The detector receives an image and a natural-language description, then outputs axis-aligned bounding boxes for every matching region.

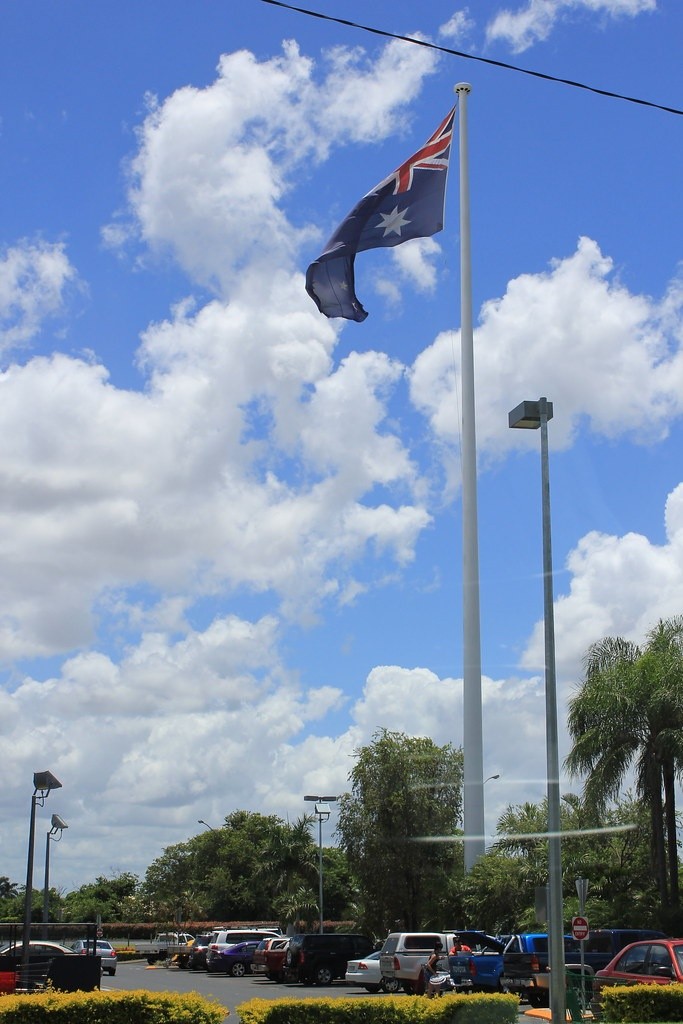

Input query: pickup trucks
[443,927,581,1001]
[251,937,298,985]
[501,930,665,1007]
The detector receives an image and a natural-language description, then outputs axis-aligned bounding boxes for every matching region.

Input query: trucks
[169,940,193,967]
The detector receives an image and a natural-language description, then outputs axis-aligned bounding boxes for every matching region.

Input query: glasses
[454,940,462,943]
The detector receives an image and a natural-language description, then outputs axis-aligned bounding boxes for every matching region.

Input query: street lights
[44,812,69,937]
[303,795,336,934]
[510,395,566,1023]
[23,770,62,991]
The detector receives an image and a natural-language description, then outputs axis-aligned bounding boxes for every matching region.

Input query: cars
[72,941,117,976]
[0,940,79,992]
[211,941,260,978]
[344,951,402,995]
[210,927,283,944]
[135,931,195,963]
[592,937,682,1005]
[187,934,214,972]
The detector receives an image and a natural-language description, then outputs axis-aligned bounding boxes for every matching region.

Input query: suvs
[256,939,289,951]
[282,935,379,987]
[380,932,498,992]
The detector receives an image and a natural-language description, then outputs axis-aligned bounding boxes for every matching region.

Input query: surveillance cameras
[52,814,69,830]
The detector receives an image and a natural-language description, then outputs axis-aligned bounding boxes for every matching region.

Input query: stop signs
[96,929,105,937]
[571,917,589,940]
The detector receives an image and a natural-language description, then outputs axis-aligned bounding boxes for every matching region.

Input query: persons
[448,937,471,995]
[424,942,445,999]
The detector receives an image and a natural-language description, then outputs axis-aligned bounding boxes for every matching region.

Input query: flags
[305,105,456,322]
